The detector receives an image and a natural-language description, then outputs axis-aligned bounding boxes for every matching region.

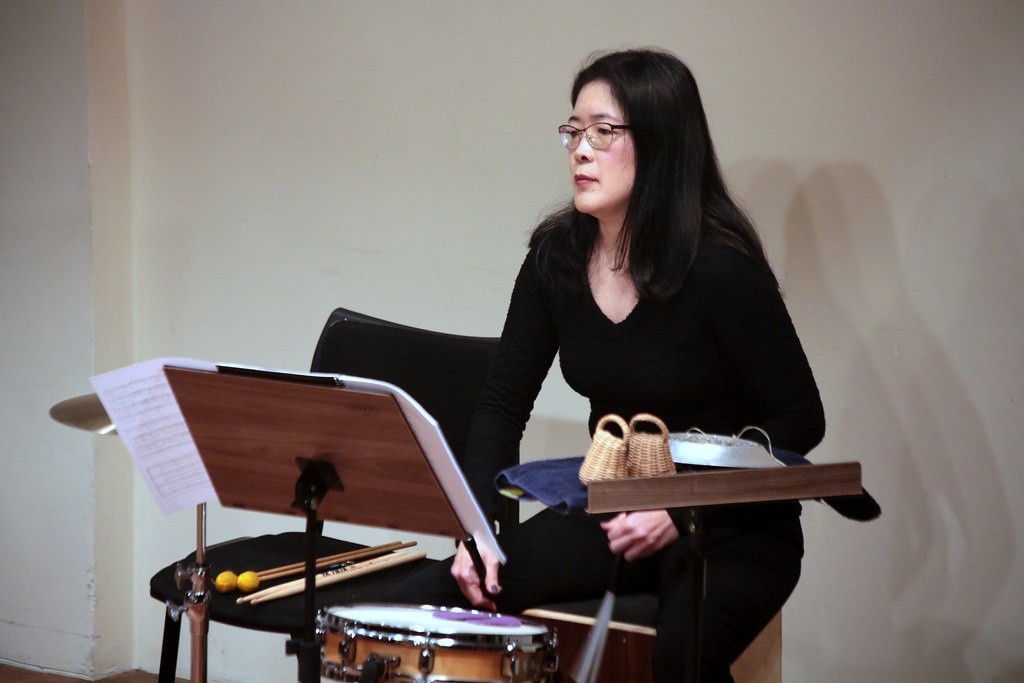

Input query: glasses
[558,123,630,150]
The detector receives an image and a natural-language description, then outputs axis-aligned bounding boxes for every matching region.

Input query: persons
[432,50,827,683]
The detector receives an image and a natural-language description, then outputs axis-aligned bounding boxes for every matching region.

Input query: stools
[523,594,784,683]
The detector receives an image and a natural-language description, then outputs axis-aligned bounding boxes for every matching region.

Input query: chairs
[150,307,501,683]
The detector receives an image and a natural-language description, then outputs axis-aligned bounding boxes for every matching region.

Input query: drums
[319,602,552,682]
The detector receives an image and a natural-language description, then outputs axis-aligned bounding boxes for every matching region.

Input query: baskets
[578,414,630,487]
[627,414,680,479]
